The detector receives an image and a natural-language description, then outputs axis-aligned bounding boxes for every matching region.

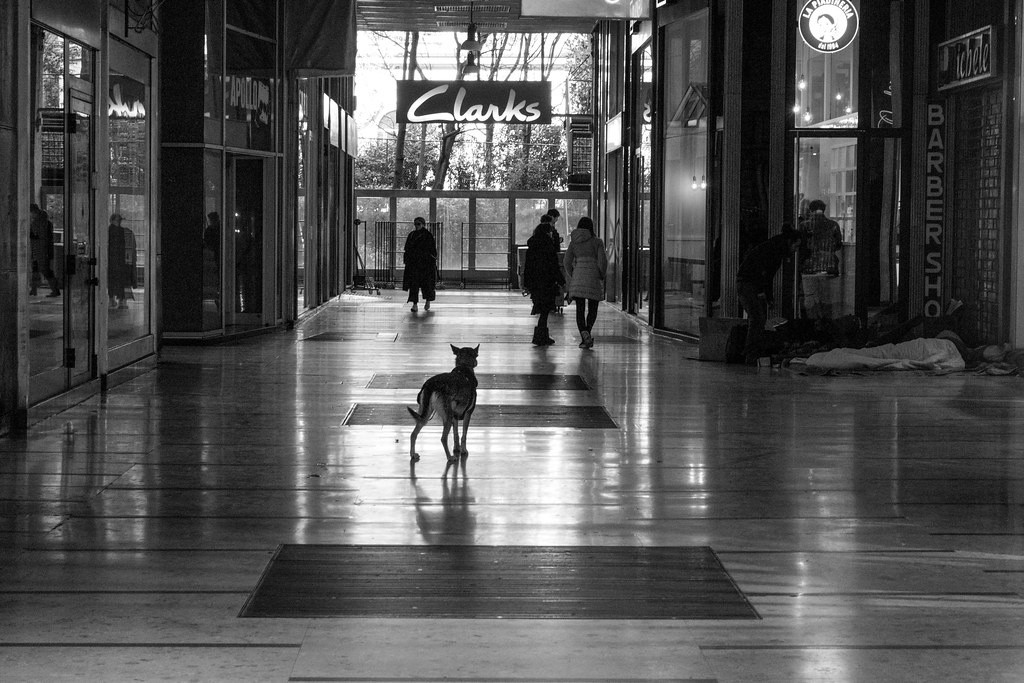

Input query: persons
[735,223,802,368]
[109,214,137,309]
[403,217,438,312]
[524,209,567,345]
[30,204,60,298]
[563,218,607,348]
[801,200,843,320]
[204,212,256,312]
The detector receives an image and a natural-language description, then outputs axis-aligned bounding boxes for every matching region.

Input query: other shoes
[46,289,62,298]
[28,288,38,295]
[424,301,431,310]
[411,304,419,312]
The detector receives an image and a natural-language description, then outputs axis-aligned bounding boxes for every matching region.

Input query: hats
[110,212,126,221]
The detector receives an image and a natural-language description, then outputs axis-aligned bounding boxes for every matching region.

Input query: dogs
[406,344,481,462]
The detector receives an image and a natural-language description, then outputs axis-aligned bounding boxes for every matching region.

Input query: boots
[532,326,556,346]
[579,341,587,348]
[580,328,594,348]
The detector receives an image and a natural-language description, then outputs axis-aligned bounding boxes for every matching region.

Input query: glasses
[414,222,422,226]
